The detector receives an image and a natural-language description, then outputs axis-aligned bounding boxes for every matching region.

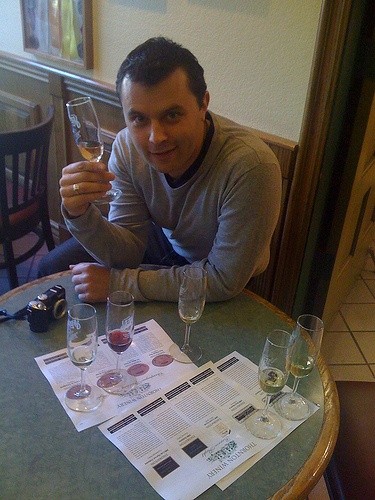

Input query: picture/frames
[20,0,94,70]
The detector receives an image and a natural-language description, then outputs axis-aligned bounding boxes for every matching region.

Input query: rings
[73,184,79,194]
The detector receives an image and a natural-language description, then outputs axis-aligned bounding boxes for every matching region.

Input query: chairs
[0,103,55,291]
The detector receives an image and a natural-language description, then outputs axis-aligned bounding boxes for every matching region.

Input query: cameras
[26,283,68,332]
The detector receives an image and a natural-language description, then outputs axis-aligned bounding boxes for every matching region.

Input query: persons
[37,37,281,303]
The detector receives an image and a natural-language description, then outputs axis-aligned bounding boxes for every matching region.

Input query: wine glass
[99,290,138,395]
[273,314,324,422]
[65,303,103,412]
[169,267,208,364]
[66,96,123,205]
[245,329,296,440]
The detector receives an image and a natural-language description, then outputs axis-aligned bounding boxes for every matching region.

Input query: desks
[0,264,340,500]
